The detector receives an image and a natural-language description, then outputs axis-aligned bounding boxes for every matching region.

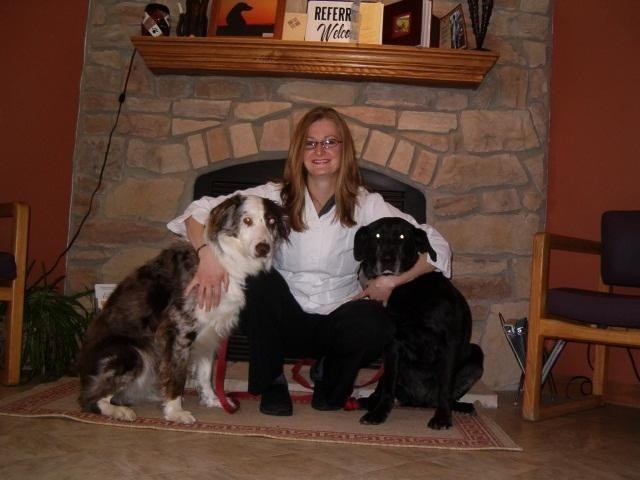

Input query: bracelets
[196,243,207,254]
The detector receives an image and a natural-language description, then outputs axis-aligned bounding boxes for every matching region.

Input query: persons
[165,104,454,416]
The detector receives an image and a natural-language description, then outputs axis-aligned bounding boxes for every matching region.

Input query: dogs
[353,216,485,431]
[76,193,294,425]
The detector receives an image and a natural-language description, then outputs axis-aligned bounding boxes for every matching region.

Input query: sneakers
[259,374,294,417]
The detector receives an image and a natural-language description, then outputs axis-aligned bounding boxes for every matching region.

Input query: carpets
[0,373,523,452]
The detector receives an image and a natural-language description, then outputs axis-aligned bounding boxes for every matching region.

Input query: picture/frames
[381,1,434,48]
[205,0,286,40]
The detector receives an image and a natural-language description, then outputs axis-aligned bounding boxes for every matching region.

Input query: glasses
[303,139,343,150]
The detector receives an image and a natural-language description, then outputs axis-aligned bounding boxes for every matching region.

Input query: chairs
[520,208,640,422]
[1,201,33,386]
